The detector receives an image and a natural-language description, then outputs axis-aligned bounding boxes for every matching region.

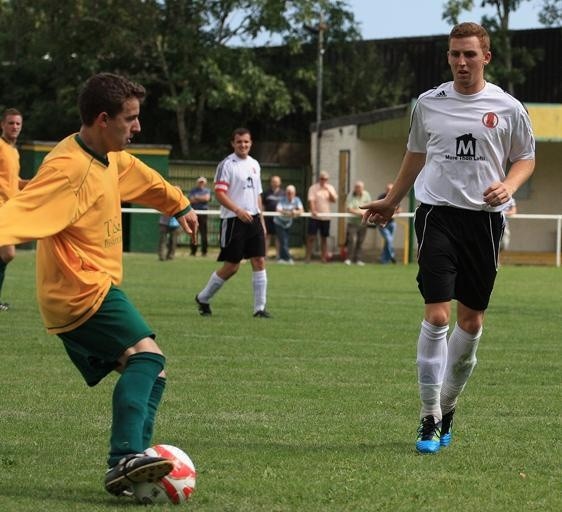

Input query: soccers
[131,444,196,505]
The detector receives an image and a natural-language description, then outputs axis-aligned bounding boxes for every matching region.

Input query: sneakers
[195,294,212,316]
[105,452,173,497]
[253,308,273,318]
[416,406,455,454]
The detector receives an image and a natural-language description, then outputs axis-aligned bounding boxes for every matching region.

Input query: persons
[188,177,211,257]
[159,183,180,262]
[262,175,285,260]
[376,182,401,265]
[342,181,371,266]
[358,23,537,455]
[304,171,337,263]
[0,73,200,498]
[194,128,273,318]
[272,184,304,264]
[0,107,30,311]
